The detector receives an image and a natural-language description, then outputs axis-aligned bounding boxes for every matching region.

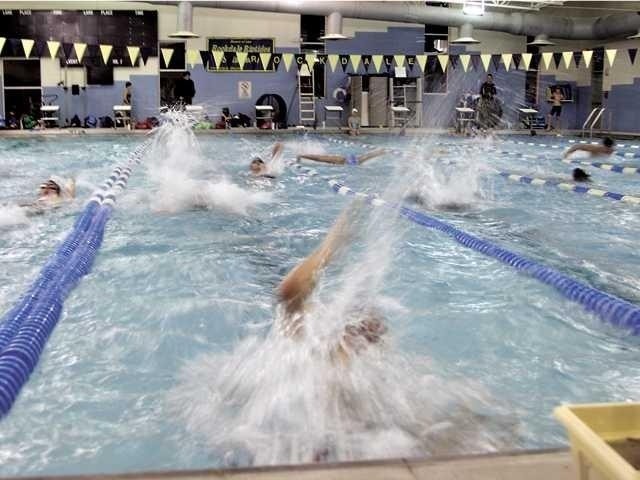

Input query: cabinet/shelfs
[351,76,423,127]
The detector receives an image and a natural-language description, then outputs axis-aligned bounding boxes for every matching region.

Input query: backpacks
[82,115,97,128]
[4,111,37,129]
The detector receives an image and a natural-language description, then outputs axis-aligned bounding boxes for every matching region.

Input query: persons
[563,137,619,159]
[458,123,495,140]
[275,211,390,364]
[241,142,285,181]
[546,87,565,132]
[296,145,398,167]
[571,169,592,183]
[123,80,132,128]
[176,72,196,105]
[348,108,362,136]
[480,74,498,101]
[18,178,77,219]
